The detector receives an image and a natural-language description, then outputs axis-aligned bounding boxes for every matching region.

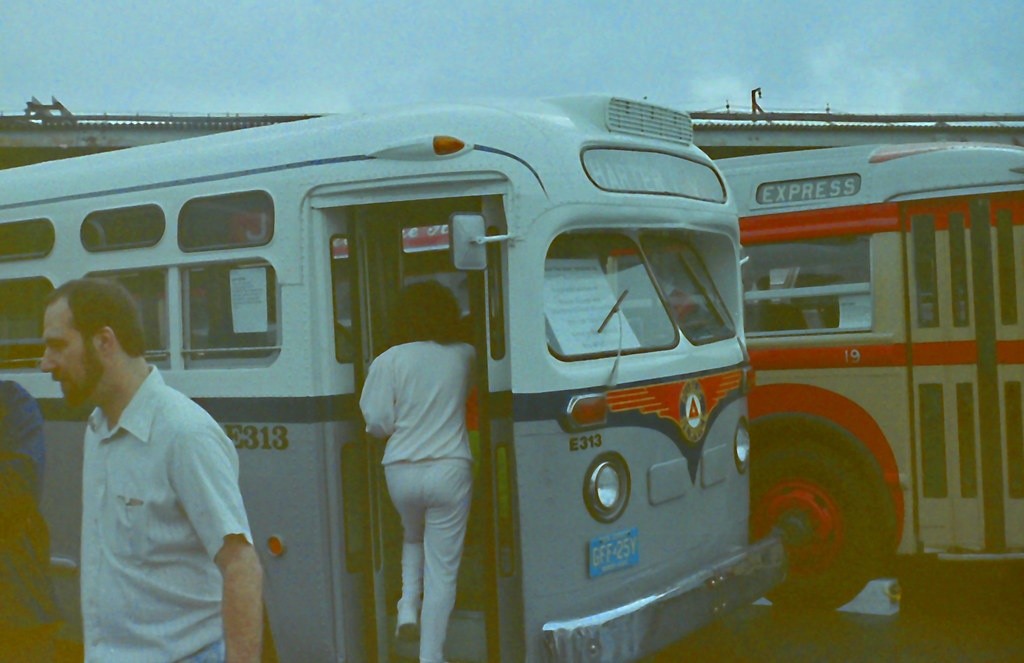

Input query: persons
[359,282,477,663]
[40,278,263,663]
[0,381,45,663]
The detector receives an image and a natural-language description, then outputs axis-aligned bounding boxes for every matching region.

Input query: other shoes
[394,622,419,644]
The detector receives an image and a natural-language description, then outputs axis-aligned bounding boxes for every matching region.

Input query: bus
[0,93,790,663]
[222,141,1024,615]
